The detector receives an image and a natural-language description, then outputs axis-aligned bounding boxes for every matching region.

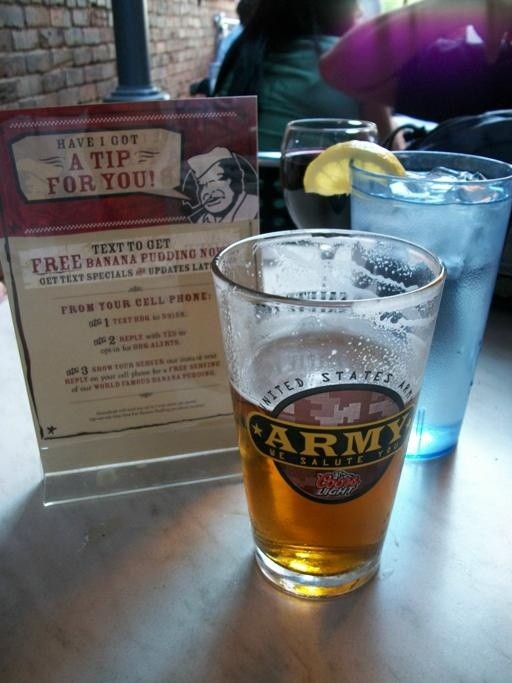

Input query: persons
[182,147,259,224]
[212,0,406,234]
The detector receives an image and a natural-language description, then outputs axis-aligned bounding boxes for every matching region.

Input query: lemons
[303,140,405,198]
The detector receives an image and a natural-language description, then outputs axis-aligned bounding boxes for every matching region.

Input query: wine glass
[280,118,379,303]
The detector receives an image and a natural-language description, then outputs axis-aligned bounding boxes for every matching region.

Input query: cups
[209,228,446,600]
[349,150,512,462]
